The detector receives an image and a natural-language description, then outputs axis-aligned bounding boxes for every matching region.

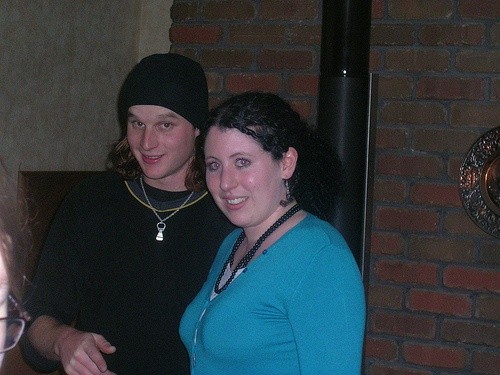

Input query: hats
[118,54,209,131]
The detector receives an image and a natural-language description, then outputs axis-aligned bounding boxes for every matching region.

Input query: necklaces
[214,204,302,293]
[140,174,194,241]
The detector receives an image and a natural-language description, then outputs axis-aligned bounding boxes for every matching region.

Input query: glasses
[0,294,31,353]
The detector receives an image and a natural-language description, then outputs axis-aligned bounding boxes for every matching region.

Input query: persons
[0,157,38,370]
[19,53,242,375]
[178,92,366,374]
